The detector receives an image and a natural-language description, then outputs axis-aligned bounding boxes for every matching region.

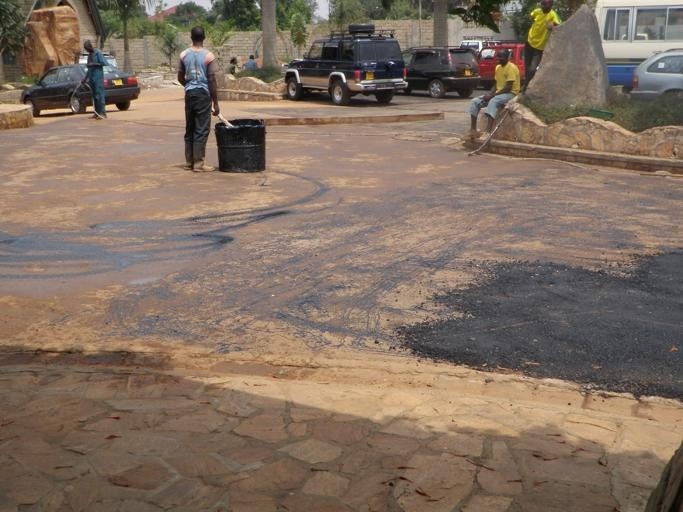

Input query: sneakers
[88,113,107,119]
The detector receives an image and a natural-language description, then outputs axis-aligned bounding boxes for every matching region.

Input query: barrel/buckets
[214,118,267,174]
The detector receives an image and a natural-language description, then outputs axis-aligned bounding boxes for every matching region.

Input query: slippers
[183,162,217,172]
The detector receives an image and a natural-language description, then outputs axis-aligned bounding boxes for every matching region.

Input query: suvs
[281,24,408,105]
[395,37,526,98]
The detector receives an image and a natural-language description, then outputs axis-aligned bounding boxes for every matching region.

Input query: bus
[594,1,683,93]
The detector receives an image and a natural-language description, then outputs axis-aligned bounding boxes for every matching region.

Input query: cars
[20,53,139,118]
[628,48,683,96]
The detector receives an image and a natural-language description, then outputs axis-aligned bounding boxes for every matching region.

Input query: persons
[177,25,221,172]
[80,40,109,120]
[521,0,563,95]
[241,54,259,70]
[223,57,238,74]
[464,47,522,139]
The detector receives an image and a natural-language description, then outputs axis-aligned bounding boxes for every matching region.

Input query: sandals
[461,132,494,141]
